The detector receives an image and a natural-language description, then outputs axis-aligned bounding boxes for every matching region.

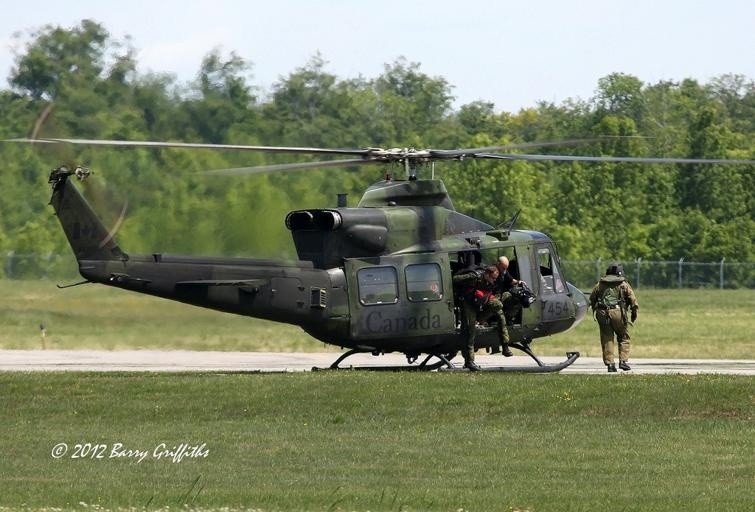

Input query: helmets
[607,262,625,275]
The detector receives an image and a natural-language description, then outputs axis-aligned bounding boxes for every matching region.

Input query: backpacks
[455,264,485,287]
[599,280,624,306]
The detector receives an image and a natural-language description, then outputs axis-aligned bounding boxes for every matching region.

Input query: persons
[585,264,639,372]
[496,256,528,325]
[453,265,514,369]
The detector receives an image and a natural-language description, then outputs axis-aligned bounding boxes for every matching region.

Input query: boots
[462,359,482,372]
[502,344,513,357]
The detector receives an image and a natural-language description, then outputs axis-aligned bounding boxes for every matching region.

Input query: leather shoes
[608,364,617,372]
[619,361,631,370]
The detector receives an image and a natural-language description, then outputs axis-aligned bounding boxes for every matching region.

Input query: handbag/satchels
[510,282,537,308]
[469,289,503,313]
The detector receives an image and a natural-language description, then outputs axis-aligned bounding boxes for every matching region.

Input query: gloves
[631,309,637,323]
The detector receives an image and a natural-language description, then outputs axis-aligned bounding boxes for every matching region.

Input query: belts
[598,306,616,310]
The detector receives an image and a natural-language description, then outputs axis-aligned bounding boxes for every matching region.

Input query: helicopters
[1,135,754,374]
